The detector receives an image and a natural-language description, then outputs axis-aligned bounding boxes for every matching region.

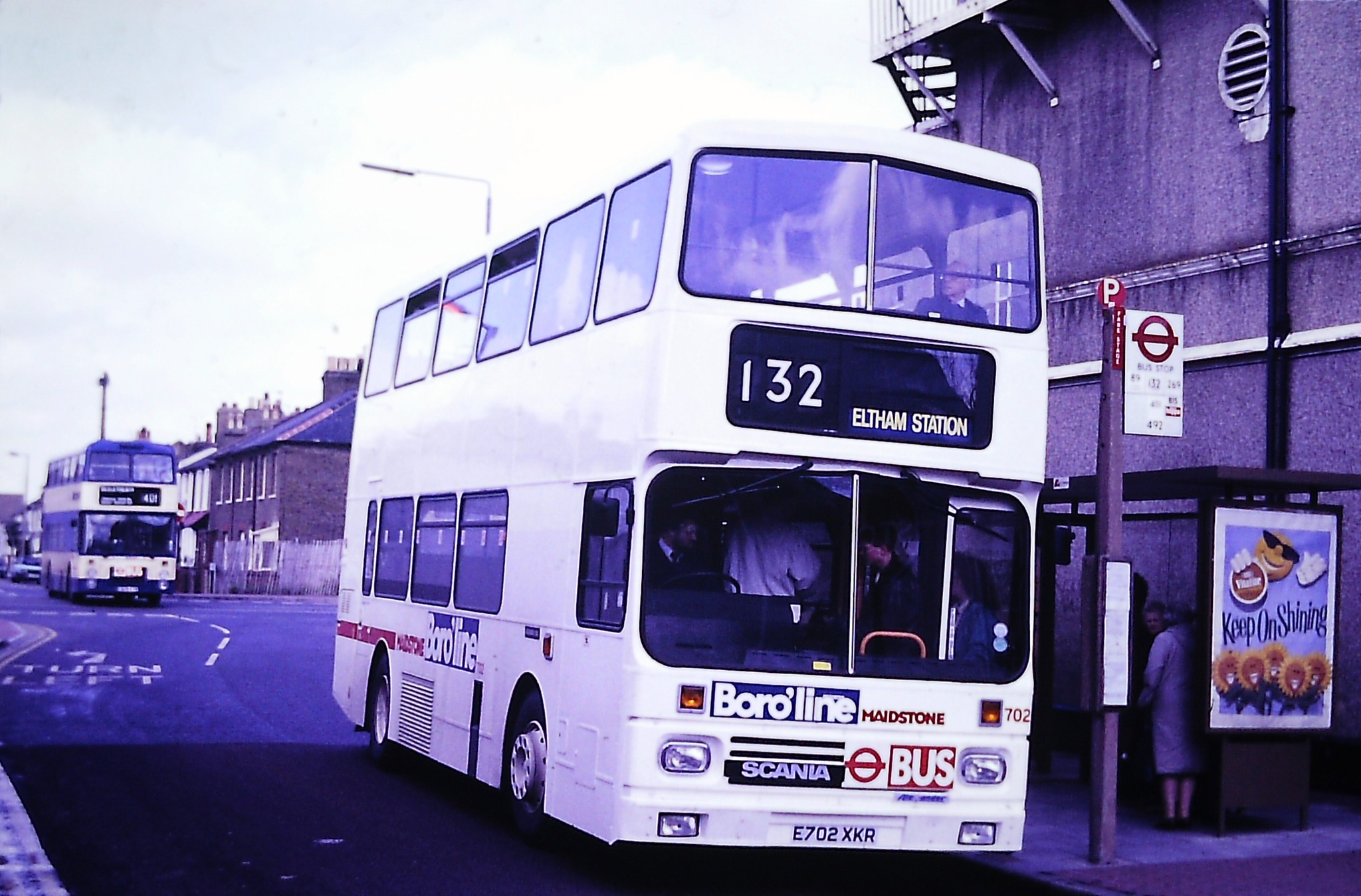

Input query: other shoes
[1155,816,1175,830]
[1176,818,1190,832]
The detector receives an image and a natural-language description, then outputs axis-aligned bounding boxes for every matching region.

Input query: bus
[331,128,1075,856]
[0,438,180,607]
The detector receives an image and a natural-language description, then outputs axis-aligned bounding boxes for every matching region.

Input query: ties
[670,552,678,563]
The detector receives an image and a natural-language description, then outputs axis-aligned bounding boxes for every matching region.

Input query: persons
[914,257,989,325]
[933,547,1000,662]
[1145,601,1205,832]
[647,510,702,590]
[721,495,821,598]
[1132,599,1170,815]
[857,520,924,656]
[1116,572,1149,810]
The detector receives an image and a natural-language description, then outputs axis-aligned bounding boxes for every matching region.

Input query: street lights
[359,161,493,239]
